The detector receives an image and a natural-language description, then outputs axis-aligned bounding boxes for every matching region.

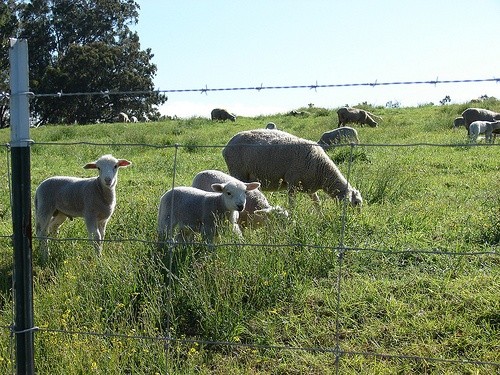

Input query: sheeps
[221,128,364,216]
[336,107,383,129]
[317,126,359,151]
[118,112,146,124]
[157,169,289,252]
[454,107,500,144]
[266,122,278,129]
[34,154,133,258]
[210,108,237,122]
